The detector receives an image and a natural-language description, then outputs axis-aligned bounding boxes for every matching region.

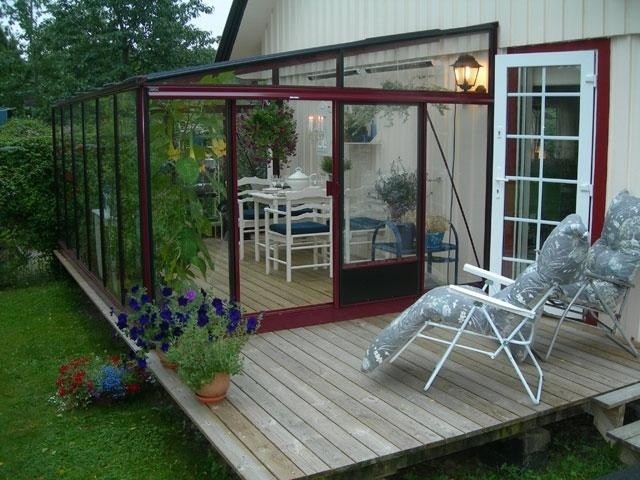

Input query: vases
[190,371,230,403]
[142,374,164,400]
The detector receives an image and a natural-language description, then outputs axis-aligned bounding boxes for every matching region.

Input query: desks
[92,208,120,294]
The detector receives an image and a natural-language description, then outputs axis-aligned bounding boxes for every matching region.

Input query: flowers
[117,284,261,372]
[54,352,144,408]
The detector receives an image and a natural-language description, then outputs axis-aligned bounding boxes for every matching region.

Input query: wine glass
[278,175,286,193]
[271,175,278,187]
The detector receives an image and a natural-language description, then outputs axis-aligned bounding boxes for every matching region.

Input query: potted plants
[407,209,453,250]
[344,104,379,142]
[374,154,444,249]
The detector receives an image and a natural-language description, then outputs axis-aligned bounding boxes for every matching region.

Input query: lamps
[449,52,488,94]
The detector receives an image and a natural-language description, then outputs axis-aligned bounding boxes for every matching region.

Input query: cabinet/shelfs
[372,216,460,287]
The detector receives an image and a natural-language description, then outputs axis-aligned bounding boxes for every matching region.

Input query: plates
[263,188,281,193]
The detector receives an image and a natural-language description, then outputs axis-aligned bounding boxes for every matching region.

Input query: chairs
[502,188,640,363]
[362,213,591,406]
[225,178,392,281]
